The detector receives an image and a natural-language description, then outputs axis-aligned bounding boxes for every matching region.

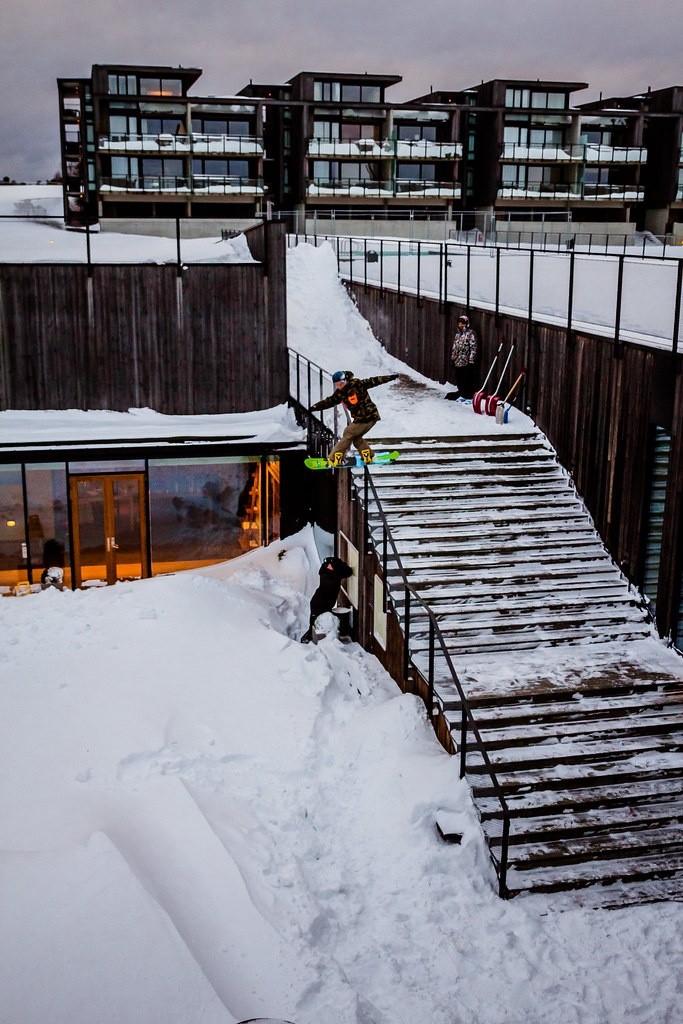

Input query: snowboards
[302,447,400,474]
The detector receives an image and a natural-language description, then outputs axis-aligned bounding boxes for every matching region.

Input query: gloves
[391,375,399,380]
[307,407,315,415]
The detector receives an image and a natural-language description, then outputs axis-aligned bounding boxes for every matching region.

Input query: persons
[305,371,400,464]
[451,315,478,397]
[300,558,354,644]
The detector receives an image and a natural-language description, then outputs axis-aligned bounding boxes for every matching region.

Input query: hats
[332,371,346,382]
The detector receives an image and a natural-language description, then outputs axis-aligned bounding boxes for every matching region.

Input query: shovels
[472,343,504,416]
[503,377,531,424]
[494,372,525,425]
[485,343,515,417]
